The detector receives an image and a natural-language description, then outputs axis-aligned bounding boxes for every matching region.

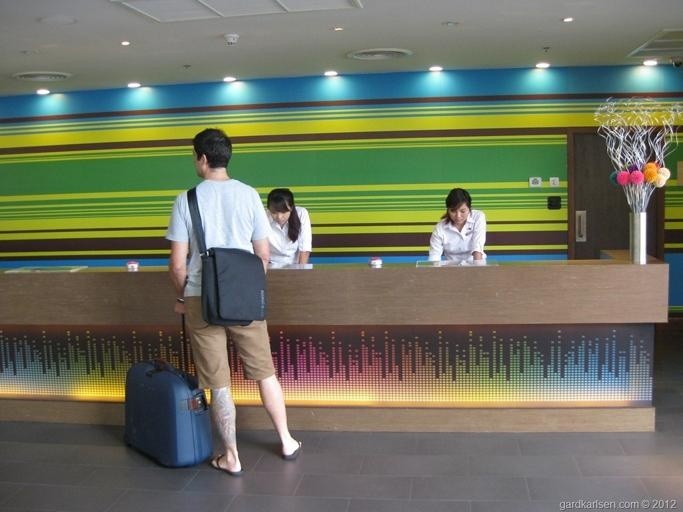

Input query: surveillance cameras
[670,57,683,67]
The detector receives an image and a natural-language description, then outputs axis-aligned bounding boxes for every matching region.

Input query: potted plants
[593,97,683,265]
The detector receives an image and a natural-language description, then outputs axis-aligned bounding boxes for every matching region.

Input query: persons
[262,188,312,265]
[427,189,488,266]
[162,127,303,477]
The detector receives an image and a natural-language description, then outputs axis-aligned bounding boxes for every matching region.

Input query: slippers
[209,454,245,477]
[282,440,303,461]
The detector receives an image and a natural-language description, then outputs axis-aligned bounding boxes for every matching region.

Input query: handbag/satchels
[200,246,267,327]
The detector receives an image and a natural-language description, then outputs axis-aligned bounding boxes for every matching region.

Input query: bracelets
[176,297,185,302]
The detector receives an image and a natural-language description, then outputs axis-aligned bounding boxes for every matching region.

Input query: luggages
[122,312,214,470]
